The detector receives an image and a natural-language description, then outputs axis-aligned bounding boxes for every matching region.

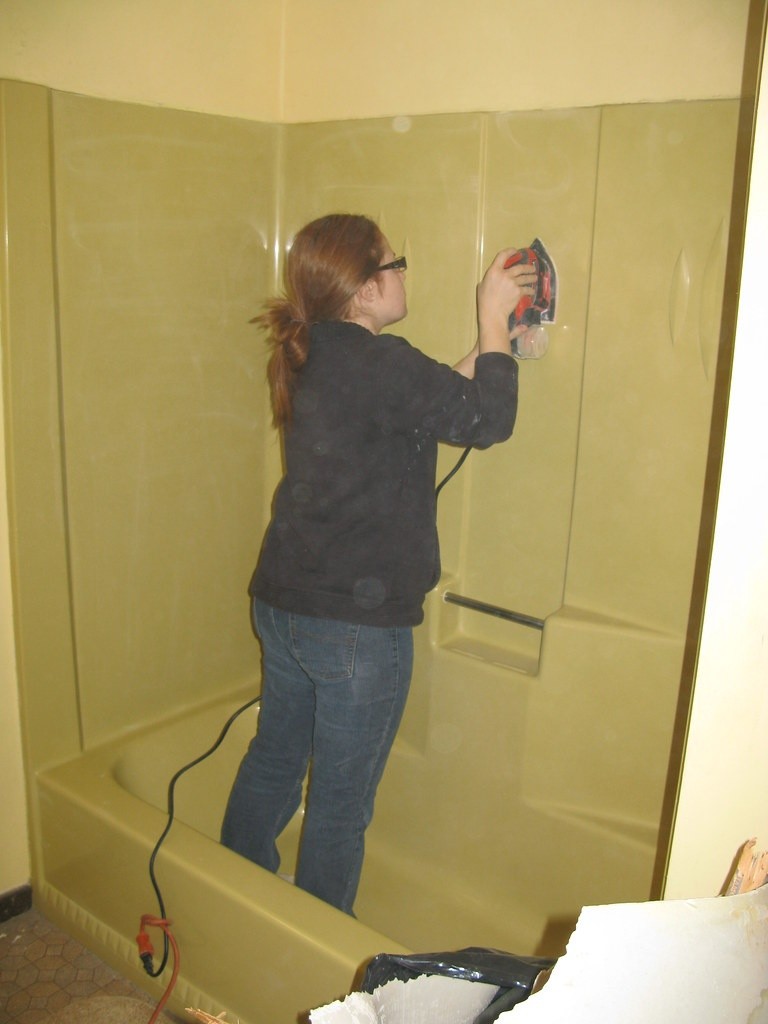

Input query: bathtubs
[33,682,662,1024]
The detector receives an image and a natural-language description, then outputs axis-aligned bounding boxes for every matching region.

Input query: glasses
[377,256,408,272]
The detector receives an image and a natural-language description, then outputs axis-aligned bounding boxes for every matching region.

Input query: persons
[219,214,536,919]
